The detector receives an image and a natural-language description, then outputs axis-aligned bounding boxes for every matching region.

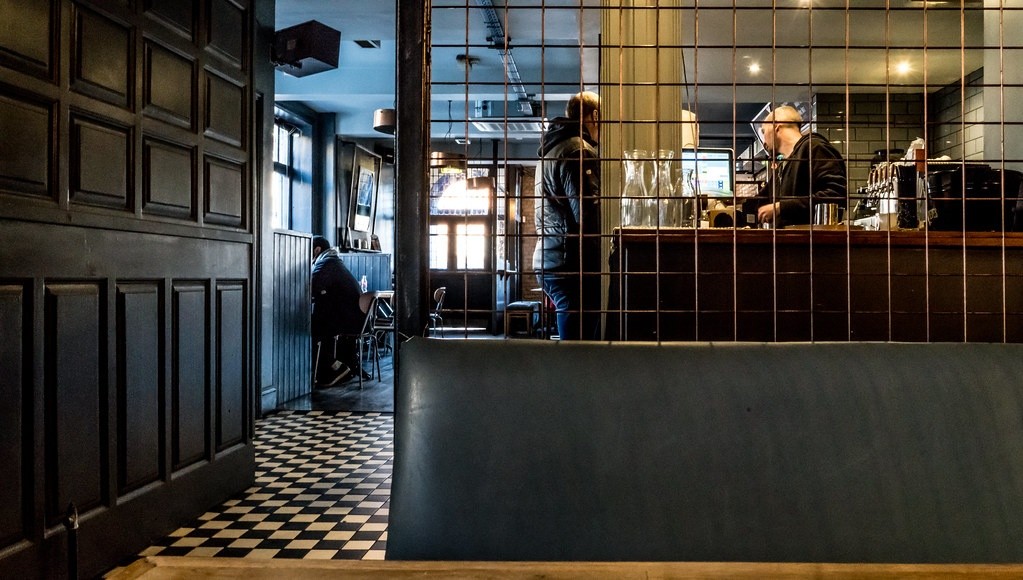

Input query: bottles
[700,210,710,228]
[621,150,694,227]
[715,200,725,209]
[361,276,368,292]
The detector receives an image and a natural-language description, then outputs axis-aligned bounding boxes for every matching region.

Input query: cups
[815,203,846,226]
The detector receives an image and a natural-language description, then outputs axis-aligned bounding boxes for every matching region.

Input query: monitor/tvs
[682,146,735,204]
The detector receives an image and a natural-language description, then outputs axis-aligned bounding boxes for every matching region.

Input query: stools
[505,301,542,339]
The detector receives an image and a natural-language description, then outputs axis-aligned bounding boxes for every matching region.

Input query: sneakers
[317,358,350,386]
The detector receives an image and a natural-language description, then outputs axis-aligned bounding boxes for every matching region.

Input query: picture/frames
[344,143,382,252]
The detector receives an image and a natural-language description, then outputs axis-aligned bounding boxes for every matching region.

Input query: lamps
[430,100,465,169]
[681,45,699,148]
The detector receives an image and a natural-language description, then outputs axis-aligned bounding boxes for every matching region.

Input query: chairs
[428,286,446,338]
[311,291,395,389]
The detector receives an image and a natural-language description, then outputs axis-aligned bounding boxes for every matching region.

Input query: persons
[311,235,371,387]
[742,106,847,229]
[532,91,601,341]
